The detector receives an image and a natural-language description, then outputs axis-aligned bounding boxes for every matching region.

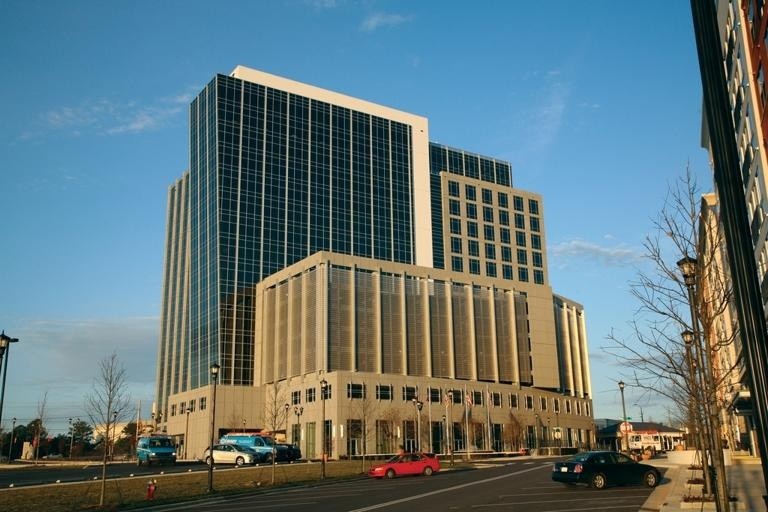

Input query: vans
[137,436,178,467]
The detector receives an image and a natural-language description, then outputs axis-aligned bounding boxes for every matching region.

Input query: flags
[443,389,493,409]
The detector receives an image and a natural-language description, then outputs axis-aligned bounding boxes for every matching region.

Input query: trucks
[628,434,661,455]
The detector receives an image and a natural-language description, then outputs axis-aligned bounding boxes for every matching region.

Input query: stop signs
[620,423,633,435]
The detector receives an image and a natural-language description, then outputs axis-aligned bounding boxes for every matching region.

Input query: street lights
[112,411,117,461]
[285,403,303,447]
[320,378,327,480]
[7,417,16,463]
[0,329,18,426]
[448,389,453,465]
[69,425,74,458]
[677,257,712,498]
[412,397,448,460]
[185,407,190,459]
[618,381,629,449]
[32,424,40,460]
[206,361,220,492]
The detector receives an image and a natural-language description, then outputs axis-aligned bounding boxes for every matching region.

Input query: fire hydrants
[148,479,156,501]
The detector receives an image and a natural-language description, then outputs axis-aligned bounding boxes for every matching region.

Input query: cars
[369,453,441,478]
[42,452,63,458]
[202,443,259,465]
[552,451,659,487]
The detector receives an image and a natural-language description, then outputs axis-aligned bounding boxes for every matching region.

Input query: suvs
[219,432,301,462]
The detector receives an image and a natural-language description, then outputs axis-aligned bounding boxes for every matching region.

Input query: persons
[396,444,404,454]
